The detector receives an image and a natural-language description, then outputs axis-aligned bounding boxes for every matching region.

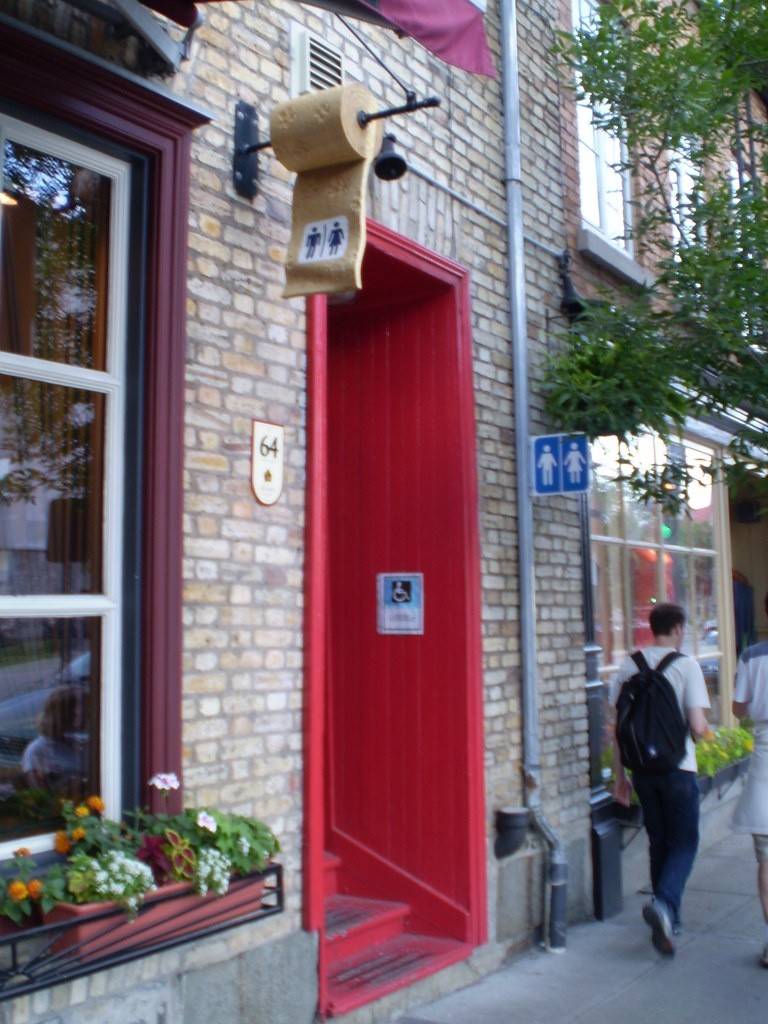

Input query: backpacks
[616,650,688,771]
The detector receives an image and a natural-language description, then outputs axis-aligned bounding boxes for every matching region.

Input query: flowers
[0,773,280,925]
[696,726,755,775]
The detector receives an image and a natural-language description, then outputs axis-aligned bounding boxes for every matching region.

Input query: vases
[691,758,754,800]
[37,861,272,965]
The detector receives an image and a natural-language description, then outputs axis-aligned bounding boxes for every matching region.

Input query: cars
[0,649,91,820]
[694,618,720,687]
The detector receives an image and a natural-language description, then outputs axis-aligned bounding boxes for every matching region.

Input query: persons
[24,689,90,793]
[608,603,711,958]
[731,591,768,967]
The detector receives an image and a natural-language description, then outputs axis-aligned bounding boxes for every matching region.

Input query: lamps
[555,250,589,320]
[375,133,406,180]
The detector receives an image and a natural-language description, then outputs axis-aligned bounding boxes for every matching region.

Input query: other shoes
[642,900,675,957]
